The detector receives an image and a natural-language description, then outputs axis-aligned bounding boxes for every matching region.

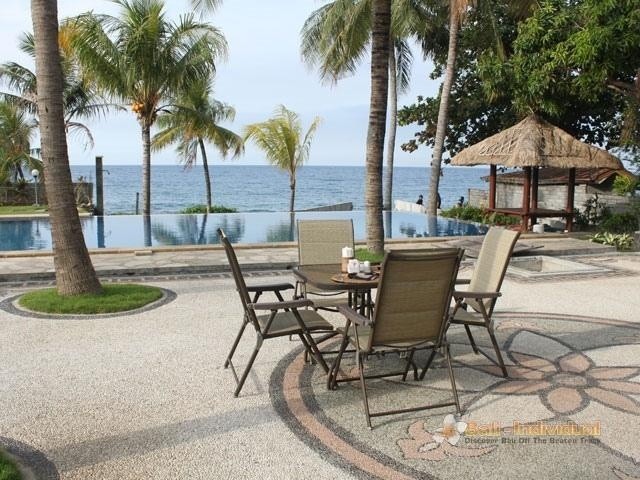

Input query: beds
[292,265,380,390]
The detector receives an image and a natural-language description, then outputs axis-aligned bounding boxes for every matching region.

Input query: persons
[437,192,442,209]
[456,196,465,208]
[416,194,423,205]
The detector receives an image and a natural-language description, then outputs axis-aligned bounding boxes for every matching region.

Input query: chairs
[419,226,521,380]
[217,227,338,397]
[327,247,465,430]
[289,219,380,365]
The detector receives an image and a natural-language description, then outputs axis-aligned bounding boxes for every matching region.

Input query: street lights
[31,168,40,207]
